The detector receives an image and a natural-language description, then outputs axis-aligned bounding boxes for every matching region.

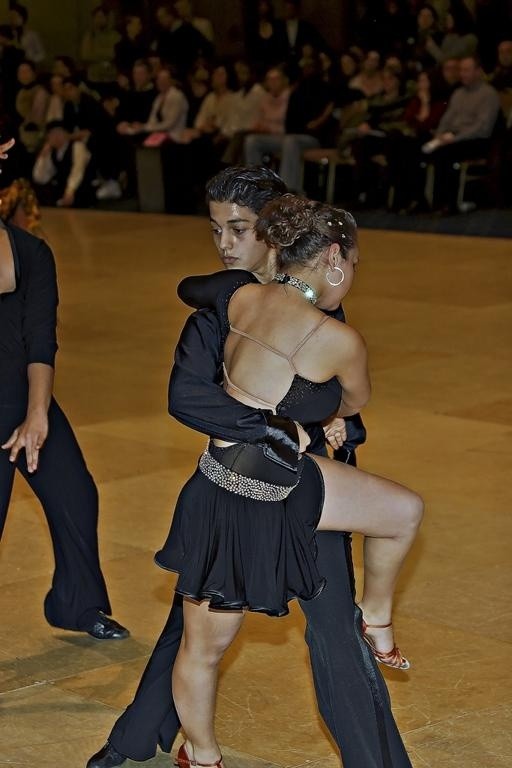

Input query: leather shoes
[86,741,127,768]
[89,614,132,641]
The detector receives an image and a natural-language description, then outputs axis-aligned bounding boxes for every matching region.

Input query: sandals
[175,741,226,768]
[357,605,411,670]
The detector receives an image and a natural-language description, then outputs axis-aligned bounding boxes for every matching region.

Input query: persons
[149,188,427,768]
[1,137,133,641]
[1,1,512,219]
[86,160,420,768]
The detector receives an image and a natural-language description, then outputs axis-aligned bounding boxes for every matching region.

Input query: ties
[156,94,169,124]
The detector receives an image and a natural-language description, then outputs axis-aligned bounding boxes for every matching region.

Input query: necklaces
[269,271,319,306]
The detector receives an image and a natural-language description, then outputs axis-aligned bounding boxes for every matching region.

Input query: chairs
[299,104,365,205]
[425,109,505,212]
